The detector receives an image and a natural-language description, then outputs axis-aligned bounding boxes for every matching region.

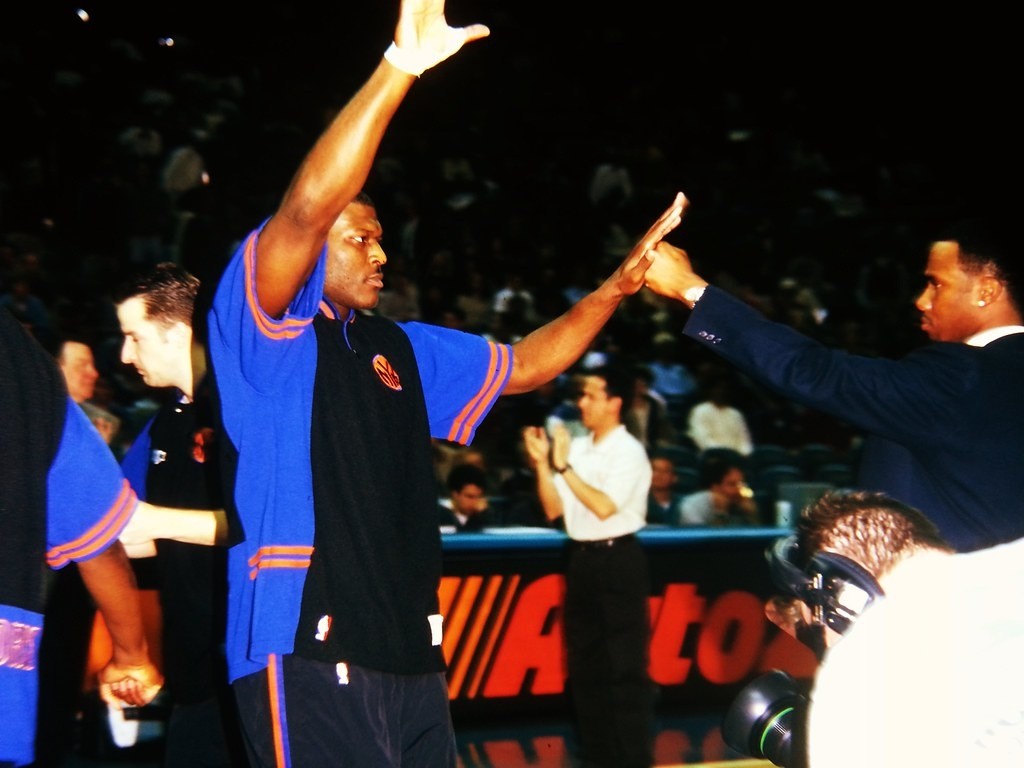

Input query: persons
[523,365,654,768]
[0,264,239,768]
[0,237,863,530]
[772,491,1024,768]
[644,220,1024,553]
[208,0,688,768]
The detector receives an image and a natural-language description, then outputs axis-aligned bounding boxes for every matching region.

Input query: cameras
[714,666,809,768]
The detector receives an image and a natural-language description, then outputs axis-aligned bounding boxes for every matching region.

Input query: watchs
[691,288,705,309]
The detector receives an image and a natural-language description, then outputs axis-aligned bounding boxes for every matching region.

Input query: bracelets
[384,41,425,76]
[559,464,573,474]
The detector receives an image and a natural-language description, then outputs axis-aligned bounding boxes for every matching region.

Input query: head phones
[770,539,886,633]
